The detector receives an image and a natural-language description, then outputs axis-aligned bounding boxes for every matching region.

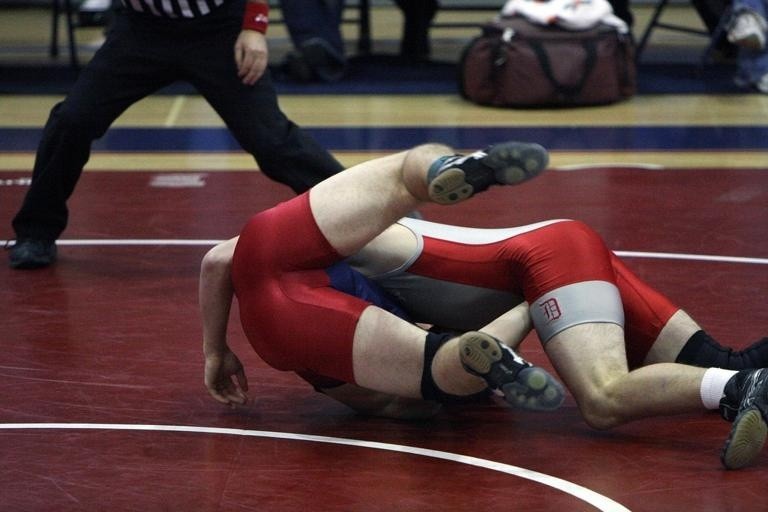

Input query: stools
[50,0,715,67]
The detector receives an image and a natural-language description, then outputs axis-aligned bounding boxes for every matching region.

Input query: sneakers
[733,73,768,92]
[727,13,768,49]
[719,368,768,471]
[459,330,566,412]
[427,141,550,205]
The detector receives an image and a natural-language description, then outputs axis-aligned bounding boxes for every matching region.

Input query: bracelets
[238,2,272,34]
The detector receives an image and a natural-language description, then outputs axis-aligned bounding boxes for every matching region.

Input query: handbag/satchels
[458,17,638,107]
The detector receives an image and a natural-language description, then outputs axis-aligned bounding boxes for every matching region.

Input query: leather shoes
[3,237,57,269]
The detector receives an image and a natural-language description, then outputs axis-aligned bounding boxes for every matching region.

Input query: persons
[237,136,566,418]
[197,215,768,468]
[9,0,346,269]
[693,0,768,95]
[277,1,439,74]
